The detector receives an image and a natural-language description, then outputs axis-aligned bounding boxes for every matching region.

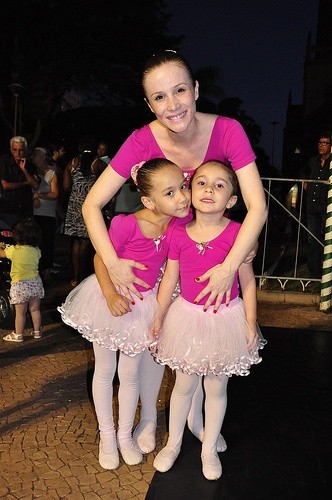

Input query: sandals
[3,331,24,342]
[31,330,42,339]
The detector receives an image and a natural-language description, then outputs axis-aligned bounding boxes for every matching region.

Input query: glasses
[317,142,331,146]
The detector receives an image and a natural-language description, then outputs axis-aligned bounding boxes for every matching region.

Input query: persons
[0,136,142,287]
[57,158,258,469]
[0,220,44,342]
[286,136,331,294]
[150,160,266,481]
[82,50,268,453]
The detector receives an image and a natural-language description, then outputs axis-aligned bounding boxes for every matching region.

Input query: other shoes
[70,280,78,286]
[199,444,221,480]
[98,436,119,470]
[152,445,180,472]
[131,417,156,453]
[202,426,226,452]
[117,432,142,465]
